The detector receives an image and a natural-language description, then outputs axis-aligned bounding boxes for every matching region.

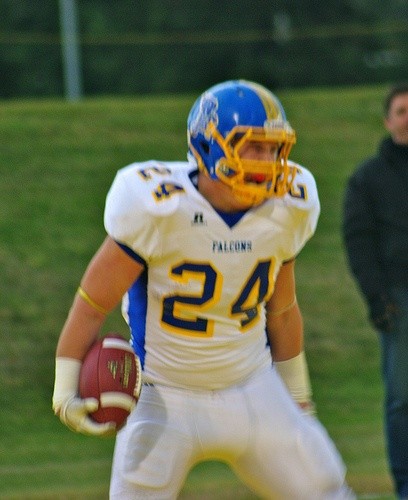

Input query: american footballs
[76,331,143,431]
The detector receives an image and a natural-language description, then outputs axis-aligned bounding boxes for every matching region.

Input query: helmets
[187,79,296,206]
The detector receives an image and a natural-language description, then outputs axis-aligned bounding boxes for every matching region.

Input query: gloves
[273,350,316,420]
[52,358,117,437]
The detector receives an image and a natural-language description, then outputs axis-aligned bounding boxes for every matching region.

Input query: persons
[340,86,408,500]
[54,82,360,500]
